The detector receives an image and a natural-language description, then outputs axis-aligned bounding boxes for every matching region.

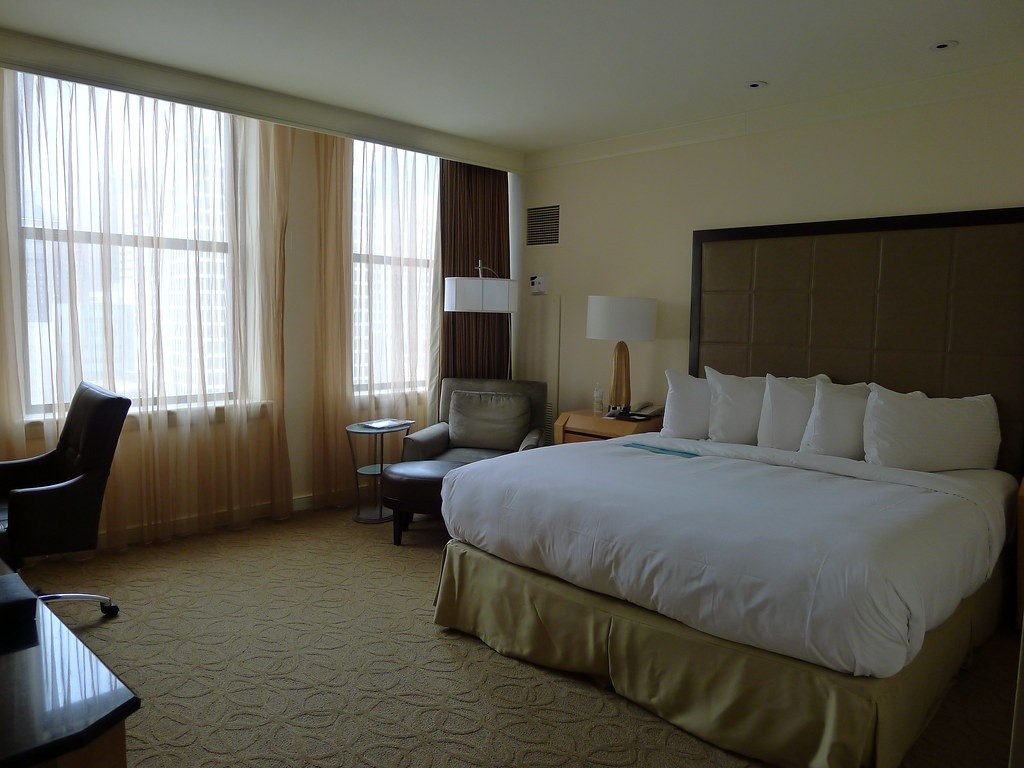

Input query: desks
[0,560,141,768]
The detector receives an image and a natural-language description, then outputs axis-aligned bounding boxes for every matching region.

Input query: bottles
[593,382,603,413]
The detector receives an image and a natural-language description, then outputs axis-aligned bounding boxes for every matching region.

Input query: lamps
[443,260,519,379]
[585,296,655,413]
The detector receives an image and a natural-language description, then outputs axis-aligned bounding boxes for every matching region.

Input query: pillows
[797,379,927,461]
[756,373,866,451]
[862,383,1000,471]
[699,365,827,445]
[658,368,764,440]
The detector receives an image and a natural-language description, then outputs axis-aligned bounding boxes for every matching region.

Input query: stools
[381,461,468,546]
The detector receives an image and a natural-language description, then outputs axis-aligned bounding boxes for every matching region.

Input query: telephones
[629,401,665,417]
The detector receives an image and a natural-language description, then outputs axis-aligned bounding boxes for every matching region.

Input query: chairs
[0,380,131,618]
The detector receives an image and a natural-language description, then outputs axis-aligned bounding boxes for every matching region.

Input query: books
[358,418,416,429]
[0,573,36,621]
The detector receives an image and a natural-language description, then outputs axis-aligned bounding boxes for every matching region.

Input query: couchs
[402,378,547,463]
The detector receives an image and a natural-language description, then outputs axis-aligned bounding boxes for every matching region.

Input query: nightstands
[554,407,664,446]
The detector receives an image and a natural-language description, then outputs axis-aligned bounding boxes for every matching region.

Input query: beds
[431,206,1024,768]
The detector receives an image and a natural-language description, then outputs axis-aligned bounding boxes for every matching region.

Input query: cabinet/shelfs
[345,419,410,524]
